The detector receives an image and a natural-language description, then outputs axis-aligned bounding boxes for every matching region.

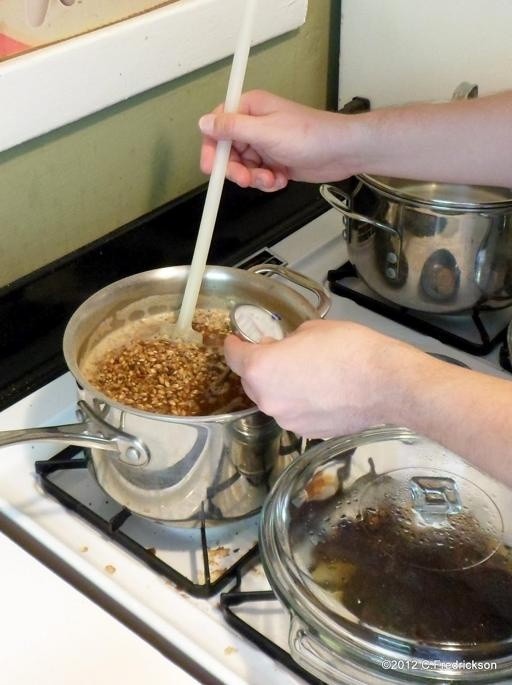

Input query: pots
[0,81,511,684]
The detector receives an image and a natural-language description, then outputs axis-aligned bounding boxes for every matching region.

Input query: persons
[199,90,512,488]
